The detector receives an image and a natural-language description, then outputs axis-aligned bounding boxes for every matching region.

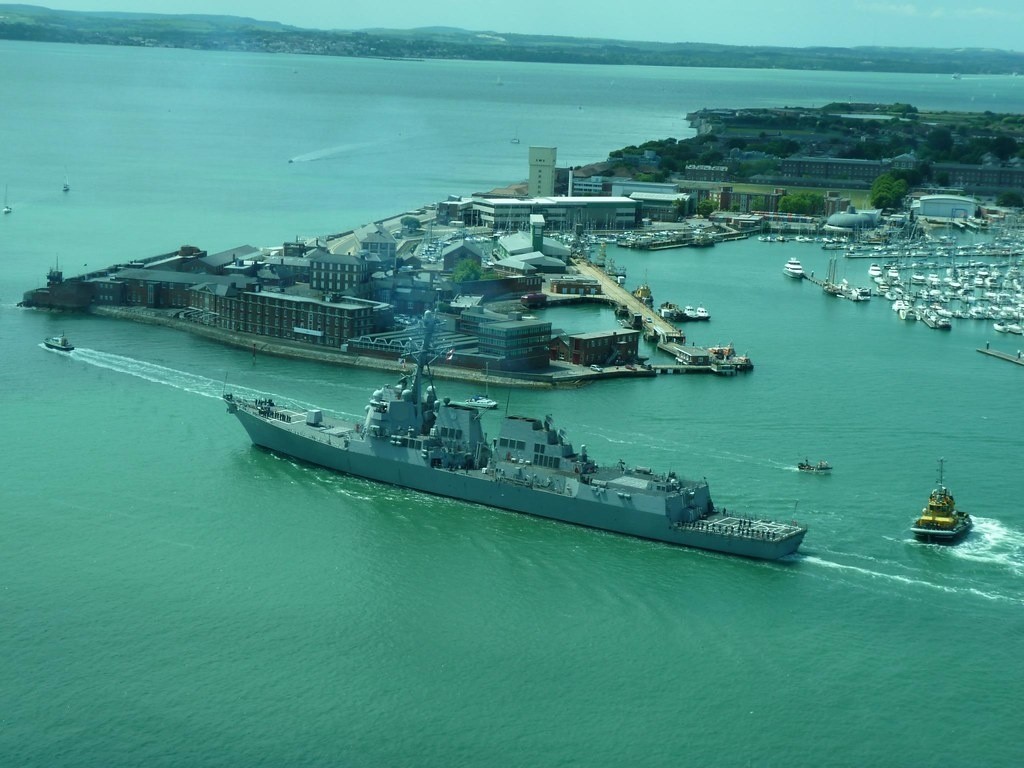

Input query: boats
[797,459,834,475]
[548,231,712,322]
[908,456,973,544]
[42,330,75,352]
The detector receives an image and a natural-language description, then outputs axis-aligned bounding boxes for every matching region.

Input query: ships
[223,308,808,562]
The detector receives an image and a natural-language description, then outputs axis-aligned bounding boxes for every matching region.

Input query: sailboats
[756,206,1024,335]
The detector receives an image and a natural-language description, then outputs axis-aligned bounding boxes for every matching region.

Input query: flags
[398,358,406,368]
[446,349,453,362]
